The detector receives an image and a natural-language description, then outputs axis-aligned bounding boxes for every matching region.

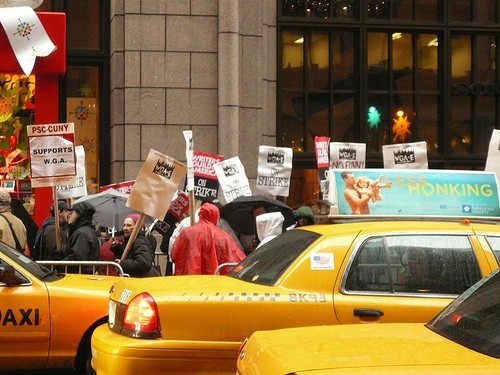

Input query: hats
[50,201,73,211]
[126,213,145,229]
[66,202,96,218]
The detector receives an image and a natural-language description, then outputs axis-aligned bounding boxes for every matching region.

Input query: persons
[0,187,26,267]
[294,206,315,226]
[171,202,246,275]
[252,206,267,245]
[8,192,196,277]
[256,211,285,249]
[341,171,373,215]
[168,196,245,273]
[355,176,392,202]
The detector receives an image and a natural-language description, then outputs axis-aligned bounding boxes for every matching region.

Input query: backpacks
[33,225,45,260]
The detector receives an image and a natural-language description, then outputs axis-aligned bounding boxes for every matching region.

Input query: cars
[236,268,499,375]
[0,240,128,375]
[91,220,500,375]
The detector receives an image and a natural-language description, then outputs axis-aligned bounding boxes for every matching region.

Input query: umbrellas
[220,194,296,246]
[69,187,154,245]
